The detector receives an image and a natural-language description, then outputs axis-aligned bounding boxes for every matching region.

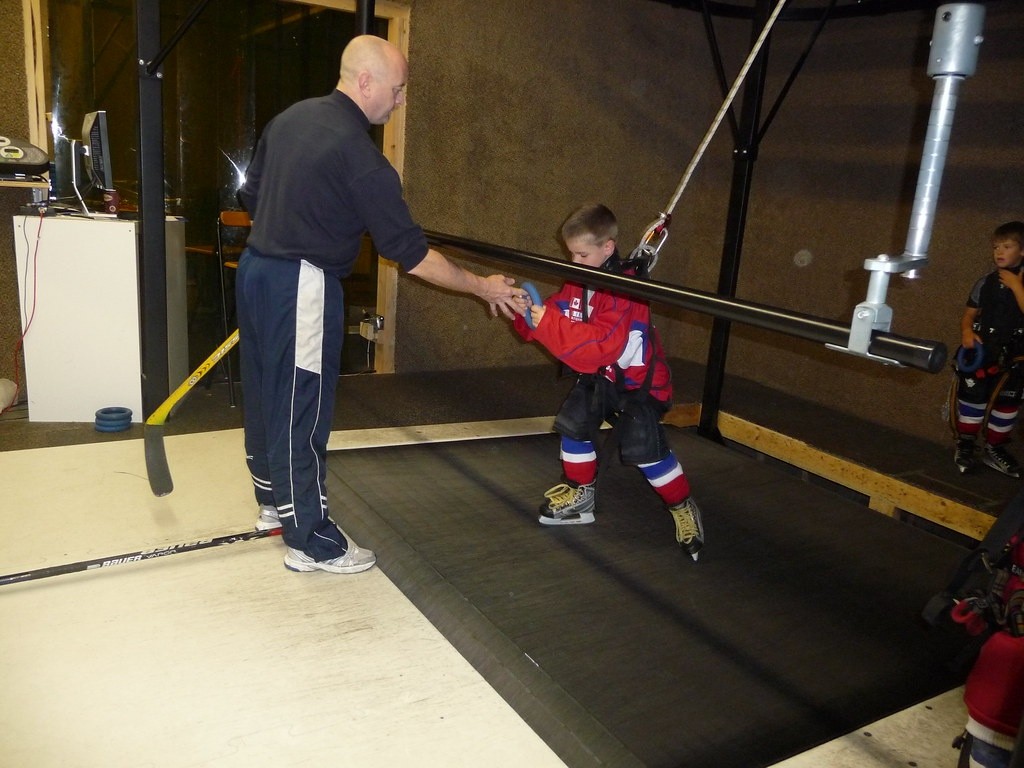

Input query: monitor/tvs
[69,110,119,218]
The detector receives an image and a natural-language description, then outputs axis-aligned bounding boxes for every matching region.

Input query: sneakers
[981,445,1024,479]
[537,477,596,526]
[955,437,979,472]
[256,505,283,531]
[282,524,376,572]
[667,499,705,562]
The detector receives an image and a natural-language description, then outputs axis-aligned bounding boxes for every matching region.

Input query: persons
[235,36,528,571]
[513,207,705,555]
[954,222,1024,476]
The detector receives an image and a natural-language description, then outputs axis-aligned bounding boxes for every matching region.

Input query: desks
[11,215,189,422]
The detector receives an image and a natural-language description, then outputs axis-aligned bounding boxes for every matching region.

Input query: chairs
[186,211,253,406]
